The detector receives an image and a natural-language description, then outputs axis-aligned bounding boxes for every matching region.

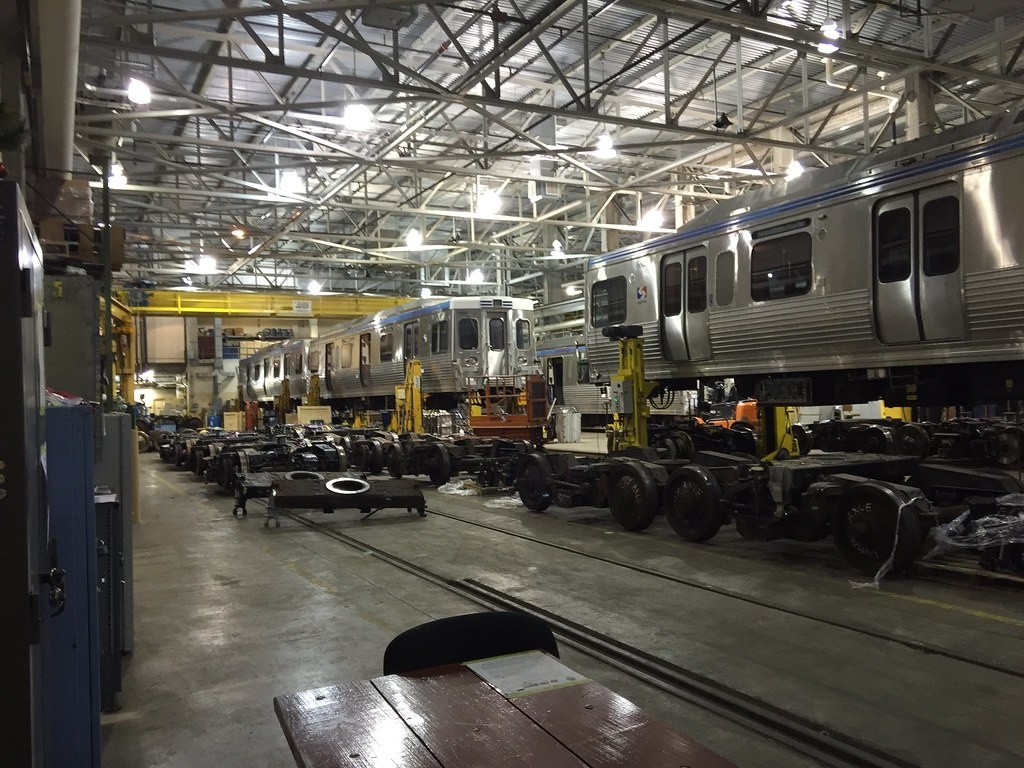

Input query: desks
[272,647,741,768]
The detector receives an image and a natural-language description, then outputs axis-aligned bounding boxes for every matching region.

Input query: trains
[237,296,547,406]
[583,106,1024,406]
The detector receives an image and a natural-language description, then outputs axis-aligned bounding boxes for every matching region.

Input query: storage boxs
[35,217,127,272]
[208,403,397,433]
[234,327,243,336]
[225,328,234,336]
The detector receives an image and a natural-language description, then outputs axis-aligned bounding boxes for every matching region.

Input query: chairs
[383,612,560,676]
